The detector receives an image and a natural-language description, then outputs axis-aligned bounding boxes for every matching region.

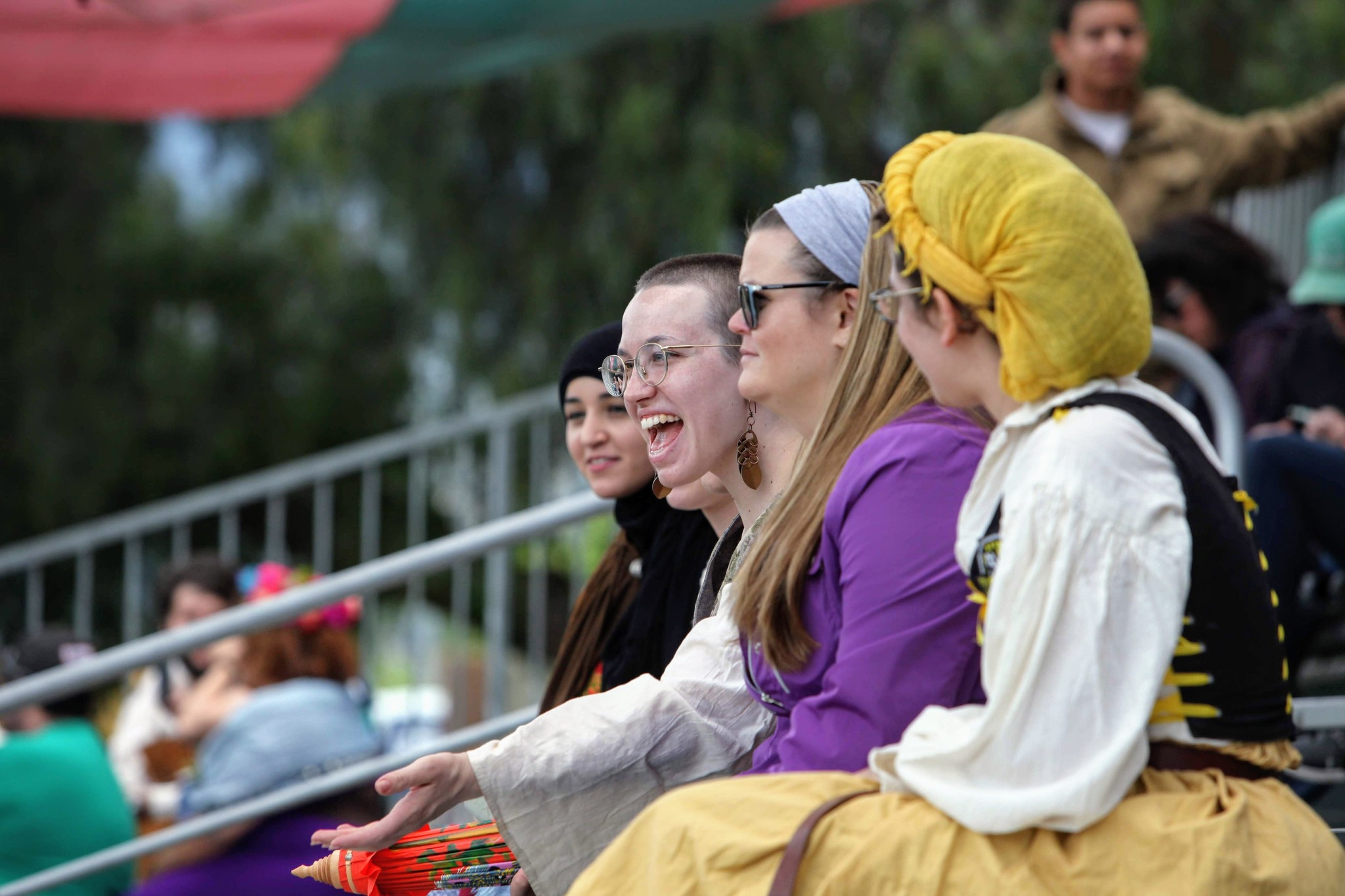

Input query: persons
[537,320,718,717]
[1131,202,1344,448]
[976,1,1345,253]
[308,251,805,896]
[0,557,382,895]
[727,177,998,777]
[1236,194,1345,675]
[566,128,1345,896]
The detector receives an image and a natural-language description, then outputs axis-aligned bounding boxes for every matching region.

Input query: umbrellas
[290,816,521,896]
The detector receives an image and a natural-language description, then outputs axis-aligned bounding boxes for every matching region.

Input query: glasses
[1151,282,1195,322]
[870,284,970,325]
[734,281,858,330]
[598,342,743,397]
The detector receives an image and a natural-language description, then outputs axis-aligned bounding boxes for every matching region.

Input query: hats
[558,321,625,417]
[1286,193,1344,305]
[237,561,360,632]
[18,634,120,699]
